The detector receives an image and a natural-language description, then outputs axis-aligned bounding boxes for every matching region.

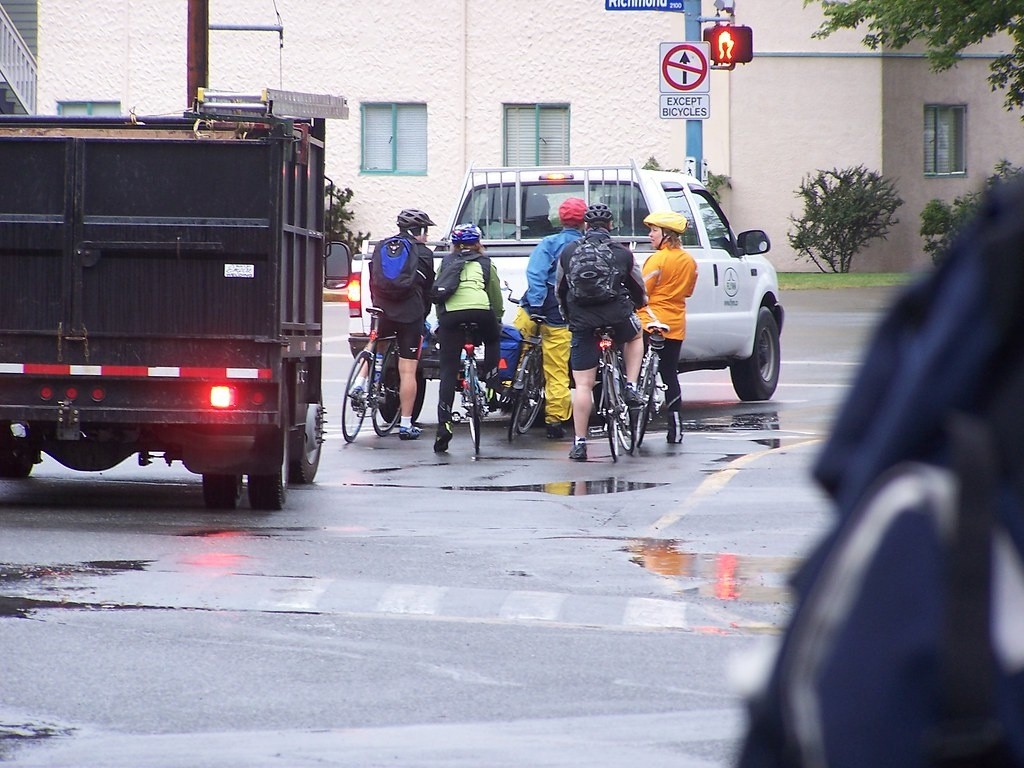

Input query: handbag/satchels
[497,323,524,380]
[428,260,465,305]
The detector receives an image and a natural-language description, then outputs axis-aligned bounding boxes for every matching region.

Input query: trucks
[0,86,352,511]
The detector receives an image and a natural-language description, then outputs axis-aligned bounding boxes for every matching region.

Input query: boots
[666,411,683,443]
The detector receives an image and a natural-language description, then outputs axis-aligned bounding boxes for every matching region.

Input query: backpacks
[564,235,620,304]
[371,236,425,300]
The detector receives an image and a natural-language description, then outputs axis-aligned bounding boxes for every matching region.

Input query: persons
[486,194,589,441]
[555,201,651,462]
[431,222,506,454]
[530,192,553,231]
[631,210,699,444]
[348,209,437,441]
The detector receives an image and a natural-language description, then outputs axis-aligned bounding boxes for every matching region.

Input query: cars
[734,174,1021,768]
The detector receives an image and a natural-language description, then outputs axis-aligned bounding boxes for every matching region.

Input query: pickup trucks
[347,160,786,401]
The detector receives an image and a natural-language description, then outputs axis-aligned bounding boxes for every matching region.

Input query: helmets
[397,209,434,228]
[643,211,687,234]
[584,204,613,222]
[451,224,481,244]
[559,198,587,226]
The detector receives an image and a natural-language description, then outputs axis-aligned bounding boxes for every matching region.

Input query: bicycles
[489,296,672,462]
[458,319,503,453]
[340,306,430,441]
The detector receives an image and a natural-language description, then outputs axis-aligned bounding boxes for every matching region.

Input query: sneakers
[485,388,499,407]
[568,442,587,461]
[624,386,646,406]
[433,423,452,452]
[546,422,568,439]
[348,386,363,398]
[399,425,421,440]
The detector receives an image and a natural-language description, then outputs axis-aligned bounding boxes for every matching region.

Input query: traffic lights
[703,25,755,69]
